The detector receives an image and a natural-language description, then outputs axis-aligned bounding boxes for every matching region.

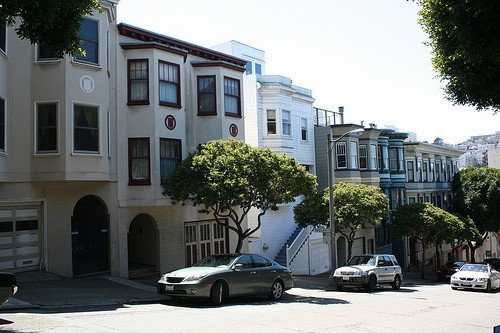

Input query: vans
[483,258,500,271]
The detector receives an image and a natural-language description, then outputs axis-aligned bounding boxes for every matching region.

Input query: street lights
[327,127,366,282]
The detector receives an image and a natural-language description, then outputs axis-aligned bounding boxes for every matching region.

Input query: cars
[450,262,500,291]
[157,252,293,304]
[436,260,469,282]
[1,271,17,306]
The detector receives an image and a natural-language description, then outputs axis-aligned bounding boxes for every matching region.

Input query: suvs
[333,253,403,291]
